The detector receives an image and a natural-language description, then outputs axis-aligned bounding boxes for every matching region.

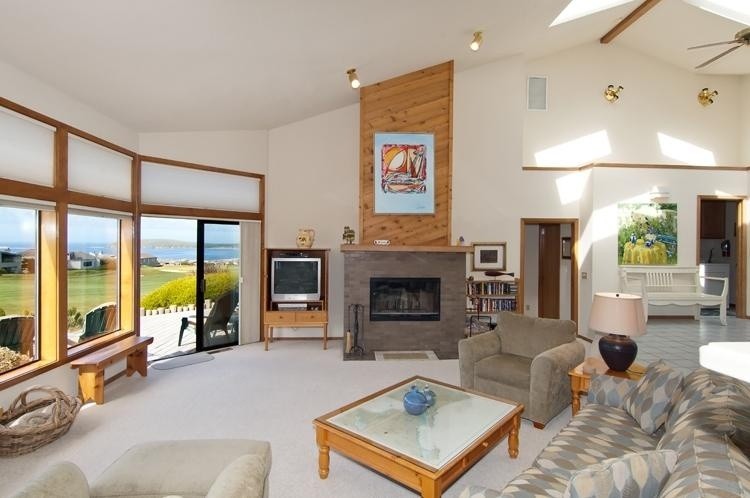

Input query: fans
[686,27,750,70]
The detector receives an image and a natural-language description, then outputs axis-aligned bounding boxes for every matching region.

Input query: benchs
[618,265,731,326]
[70,335,154,404]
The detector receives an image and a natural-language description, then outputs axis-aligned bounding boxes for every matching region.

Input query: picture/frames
[471,241,507,271]
[561,237,571,259]
[372,130,436,217]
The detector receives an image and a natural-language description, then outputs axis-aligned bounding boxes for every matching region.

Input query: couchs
[458,357,750,498]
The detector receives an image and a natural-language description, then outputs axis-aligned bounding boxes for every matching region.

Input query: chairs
[6,438,272,498]
[0,314,35,364]
[178,288,239,350]
[66,302,118,349]
[458,311,586,430]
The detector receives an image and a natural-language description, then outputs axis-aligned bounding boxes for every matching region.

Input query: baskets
[0,386,82,457]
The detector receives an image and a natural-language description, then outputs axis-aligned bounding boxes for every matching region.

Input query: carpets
[373,350,439,361]
[147,351,215,370]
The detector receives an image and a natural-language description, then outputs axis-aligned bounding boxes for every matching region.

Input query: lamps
[469,31,483,51]
[604,84,624,104]
[347,68,360,90]
[590,292,647,373]
[698,87,717,107]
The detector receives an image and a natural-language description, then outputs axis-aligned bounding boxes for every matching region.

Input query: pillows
[623,359,692,436]
[563,449,679,497]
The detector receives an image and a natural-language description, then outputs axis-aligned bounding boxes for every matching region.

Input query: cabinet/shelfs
[263,248,331,350]
[465,280,519,337]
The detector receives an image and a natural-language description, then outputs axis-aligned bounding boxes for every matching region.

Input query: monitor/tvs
[269,256,322,303]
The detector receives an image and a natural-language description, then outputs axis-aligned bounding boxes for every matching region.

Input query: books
[466,282,518,313]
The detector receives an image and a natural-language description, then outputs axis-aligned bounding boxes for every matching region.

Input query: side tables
[568,357,648,417]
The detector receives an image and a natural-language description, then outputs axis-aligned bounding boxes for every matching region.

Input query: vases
[296,228,315,249]
